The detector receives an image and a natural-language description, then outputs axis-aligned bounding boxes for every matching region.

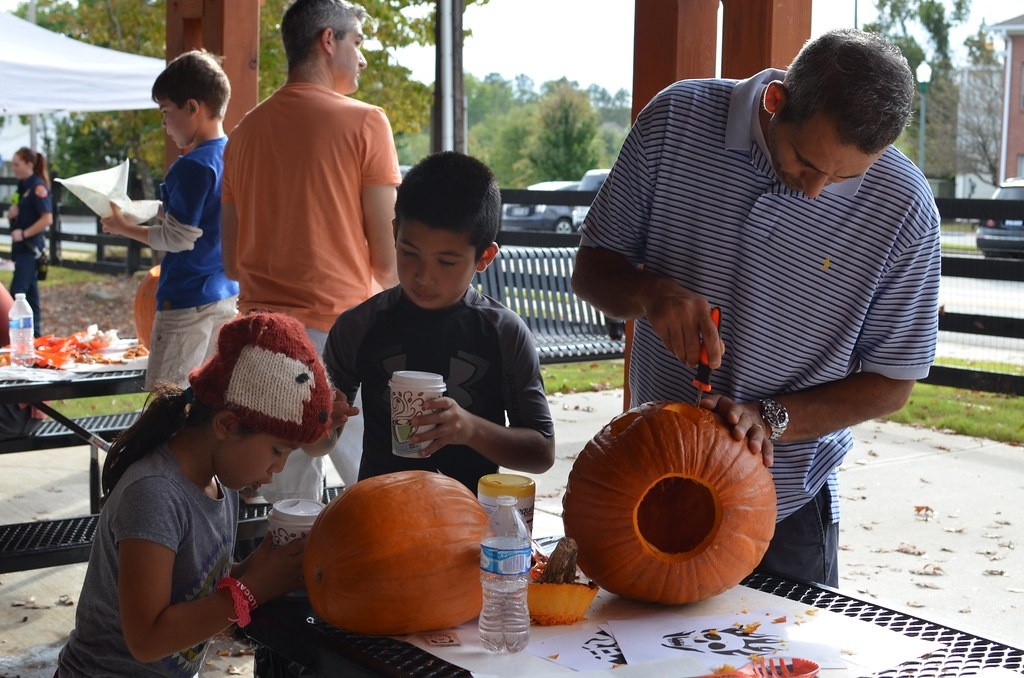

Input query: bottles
[478,497,530,654]
[8,293,36,371]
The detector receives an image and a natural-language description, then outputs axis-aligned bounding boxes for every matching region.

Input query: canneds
[477,474,535,537]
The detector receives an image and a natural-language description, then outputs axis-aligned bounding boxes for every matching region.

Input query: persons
[99,50,239,407]
[302,150,556,498]
[3,146,54,338]
[569,27,941,588]
[222,1,401,509]
[50,312,333,678]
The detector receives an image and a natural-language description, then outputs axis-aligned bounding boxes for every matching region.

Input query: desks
[235,508,1024,678]
[0,339,148,515]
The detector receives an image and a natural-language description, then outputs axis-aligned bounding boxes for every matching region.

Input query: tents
[1,4,165,116]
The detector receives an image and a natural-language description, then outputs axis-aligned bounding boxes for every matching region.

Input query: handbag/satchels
[36,251,47,281]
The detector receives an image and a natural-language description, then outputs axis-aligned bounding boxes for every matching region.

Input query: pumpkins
[134,265,160,352]
[303,469,493,636]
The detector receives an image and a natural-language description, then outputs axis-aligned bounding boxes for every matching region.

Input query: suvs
[975,177,1024,259]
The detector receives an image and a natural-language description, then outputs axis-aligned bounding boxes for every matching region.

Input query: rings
[754,424,763,428]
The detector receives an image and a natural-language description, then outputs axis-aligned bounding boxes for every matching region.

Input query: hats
[189,313,333,444]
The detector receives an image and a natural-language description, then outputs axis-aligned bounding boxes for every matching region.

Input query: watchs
[754,398,789,442]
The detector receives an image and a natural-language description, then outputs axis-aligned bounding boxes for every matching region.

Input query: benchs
[0,516,100,575]
[469,248,627,365]
[0,412,142,455]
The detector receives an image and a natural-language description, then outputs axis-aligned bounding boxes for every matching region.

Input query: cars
[501,180,579,234]
[572,170,613,230]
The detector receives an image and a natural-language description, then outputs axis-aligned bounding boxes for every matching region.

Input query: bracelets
[215,578,250,628]
[21,230,26,240]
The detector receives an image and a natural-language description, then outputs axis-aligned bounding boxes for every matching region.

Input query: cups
[266,499,327,598]
[388,371,447,458]
[478,475,536,541]
